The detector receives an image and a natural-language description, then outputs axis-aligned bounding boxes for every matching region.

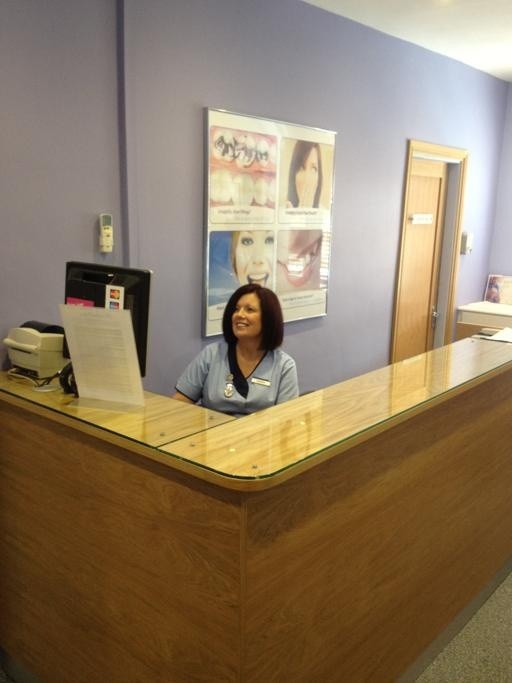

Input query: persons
[276,230,321,291]
[231,229,274,291]
[173,285,299,417]
[285,139,321,210]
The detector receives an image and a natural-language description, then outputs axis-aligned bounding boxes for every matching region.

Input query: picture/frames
[202,107,337,339]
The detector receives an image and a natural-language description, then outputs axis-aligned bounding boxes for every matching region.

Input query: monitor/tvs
[60,261,152,396]
